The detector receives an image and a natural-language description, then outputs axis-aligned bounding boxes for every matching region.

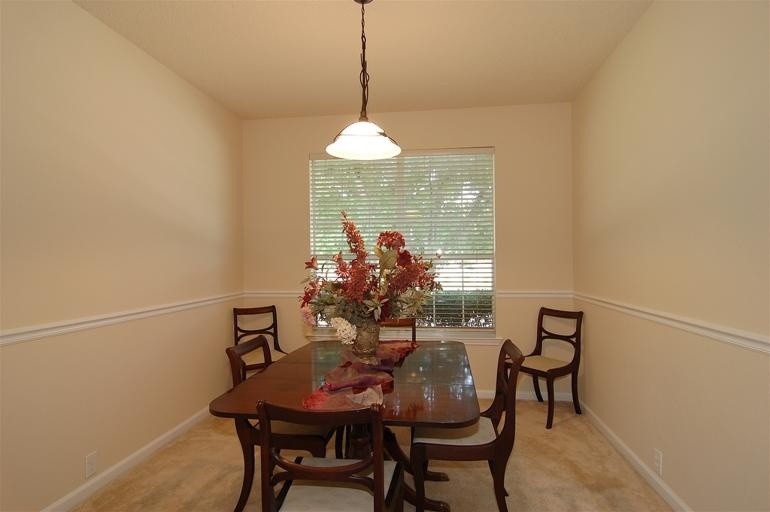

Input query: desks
[210,339,481,511]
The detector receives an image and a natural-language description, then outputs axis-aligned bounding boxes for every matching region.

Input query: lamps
[323,0,403,162]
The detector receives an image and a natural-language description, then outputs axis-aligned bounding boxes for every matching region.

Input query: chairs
[225,334,346,512]
[233,304,289,372]
[501,306,585,429]
[298,209,446,346]
[409,337,527,510]
[258,402,402,511]
